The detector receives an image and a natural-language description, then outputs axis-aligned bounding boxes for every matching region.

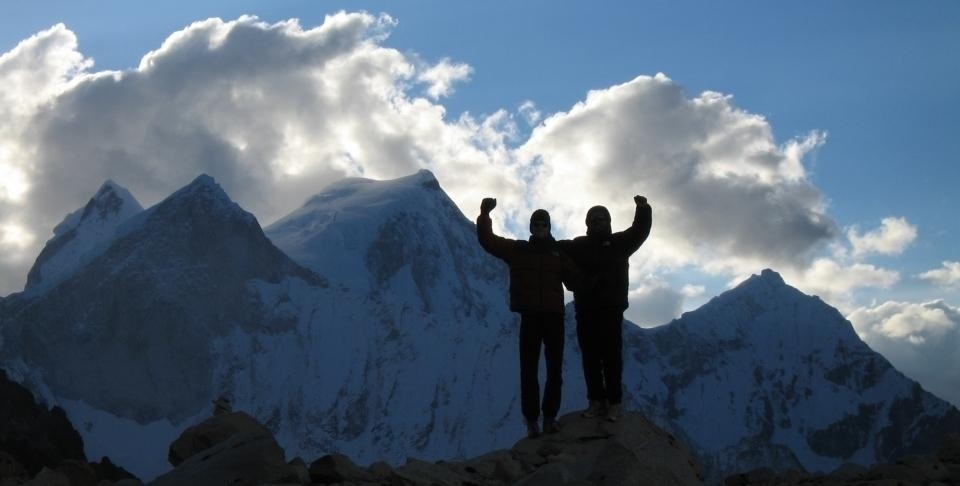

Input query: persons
[474,195,574,438]
[549,195,653,422]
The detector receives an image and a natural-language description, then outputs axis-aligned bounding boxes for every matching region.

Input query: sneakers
[526,420,541,439]
[579,397,625,423]
[543,418,561,434]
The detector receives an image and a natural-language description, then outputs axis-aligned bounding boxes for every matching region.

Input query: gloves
[480,197,497,214]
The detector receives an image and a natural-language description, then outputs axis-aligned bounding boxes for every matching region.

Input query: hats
[529,209,550,222]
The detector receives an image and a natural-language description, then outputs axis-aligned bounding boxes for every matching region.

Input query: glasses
[533,223,549,227]
